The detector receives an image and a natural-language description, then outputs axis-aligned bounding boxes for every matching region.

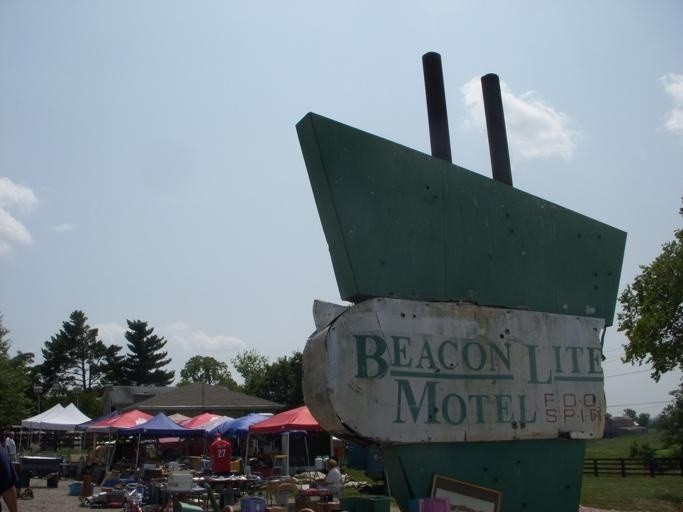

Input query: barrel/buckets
[68,483,82,495]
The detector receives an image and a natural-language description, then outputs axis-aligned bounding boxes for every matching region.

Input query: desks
[159,487,208,512]
[192,478,260,512]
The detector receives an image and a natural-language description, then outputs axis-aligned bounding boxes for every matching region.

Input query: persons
[207,431,233,504]
[29,437,41,455]
[0,444,20,512]
[315,456,343,497]
[3,430,16,461]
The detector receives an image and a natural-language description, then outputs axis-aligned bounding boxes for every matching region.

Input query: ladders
[270,455,288,475]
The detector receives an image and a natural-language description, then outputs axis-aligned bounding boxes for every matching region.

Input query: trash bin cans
[340,497,392,512]
